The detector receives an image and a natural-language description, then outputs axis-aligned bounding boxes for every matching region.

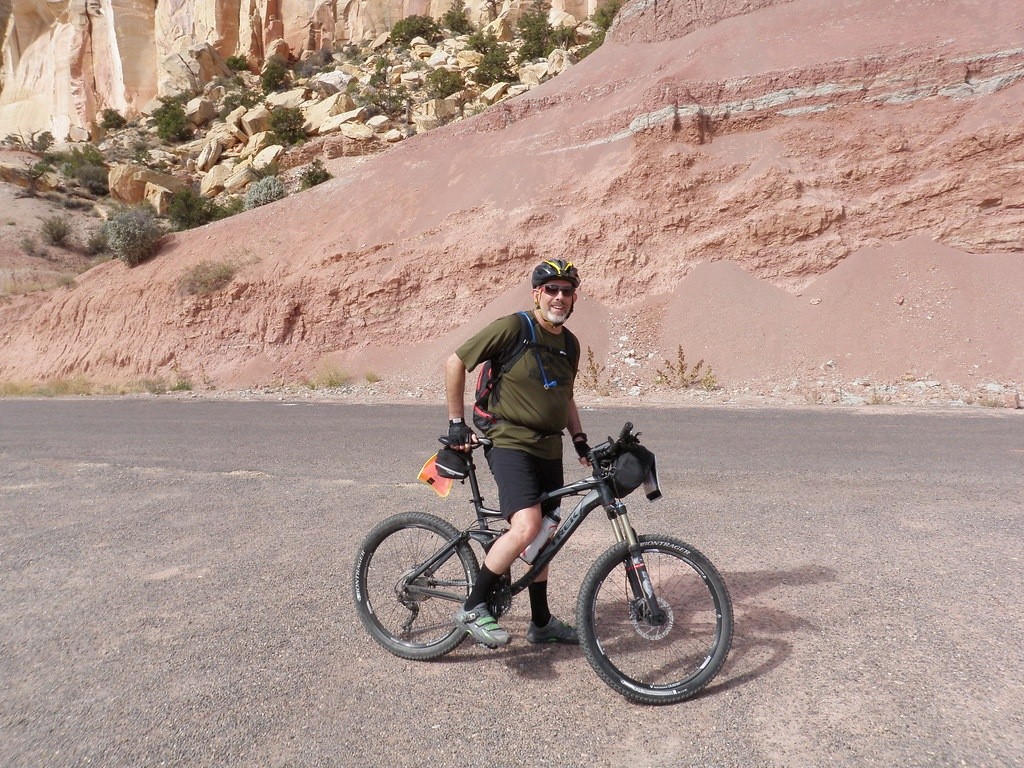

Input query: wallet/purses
[435,448,469,479]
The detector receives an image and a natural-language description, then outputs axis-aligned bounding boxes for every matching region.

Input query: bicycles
[349,422,736,707]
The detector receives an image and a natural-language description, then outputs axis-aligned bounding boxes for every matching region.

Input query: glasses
[540,285,576,297]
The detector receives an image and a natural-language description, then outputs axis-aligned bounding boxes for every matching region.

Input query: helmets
[532,259,581,289]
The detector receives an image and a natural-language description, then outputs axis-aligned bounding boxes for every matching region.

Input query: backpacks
[472,311,537,433]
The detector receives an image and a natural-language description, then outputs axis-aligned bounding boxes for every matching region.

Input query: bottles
[517,506,562,563]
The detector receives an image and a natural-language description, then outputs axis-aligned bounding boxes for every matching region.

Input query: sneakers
[525,614,580,646]
[451,602,512,648]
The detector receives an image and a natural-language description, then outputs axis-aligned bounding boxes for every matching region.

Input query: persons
[446,259,592,647]
